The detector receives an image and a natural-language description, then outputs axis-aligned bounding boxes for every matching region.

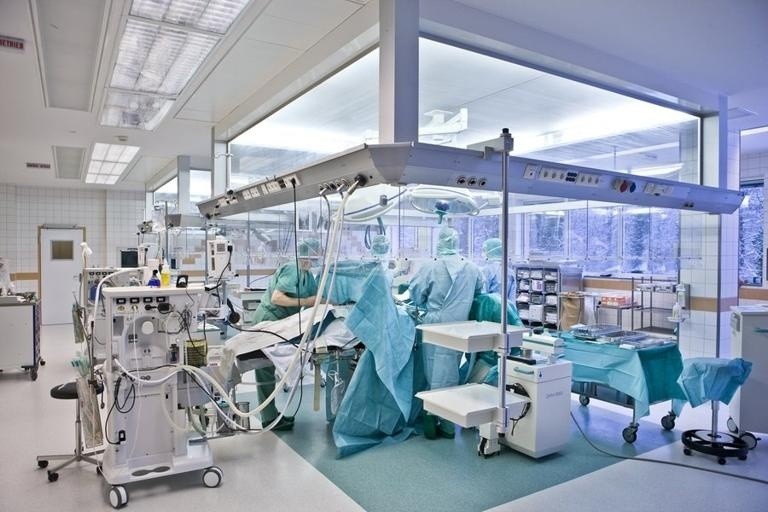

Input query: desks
[0,303,40,380]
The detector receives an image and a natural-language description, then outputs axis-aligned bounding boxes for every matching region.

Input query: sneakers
[262,413,295,429]
[425,425,455,440]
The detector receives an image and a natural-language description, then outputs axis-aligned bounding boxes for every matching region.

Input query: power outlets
[217,174,302,208]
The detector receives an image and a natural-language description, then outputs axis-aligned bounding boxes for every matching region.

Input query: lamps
[79,241,93,269]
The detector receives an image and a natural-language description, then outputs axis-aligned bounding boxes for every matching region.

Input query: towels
[76,377,103,448]
[72,304,83,344]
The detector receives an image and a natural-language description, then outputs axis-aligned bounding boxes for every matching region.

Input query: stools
[36,381,108,482]
[681,356,750,466]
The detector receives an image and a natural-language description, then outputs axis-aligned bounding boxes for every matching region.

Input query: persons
[372,236,390,254]
[251,237,329,431]
[481,238,514,300]
[409,228,486,440]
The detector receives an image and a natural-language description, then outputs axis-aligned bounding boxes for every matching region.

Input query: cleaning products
[147,270,162,286]
[161,258,171,287]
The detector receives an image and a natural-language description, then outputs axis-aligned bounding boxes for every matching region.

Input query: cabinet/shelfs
[630,277,679,351]
[137,230,160,268]
[513,264,583,335]
[557,290,598,333]
[596,302,642,330]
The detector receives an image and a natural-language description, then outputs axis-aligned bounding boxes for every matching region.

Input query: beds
[225,304,410,423]
[528,327,684,444]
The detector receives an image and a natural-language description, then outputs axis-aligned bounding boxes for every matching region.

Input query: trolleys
[725,303,768,452]
[0,289,47,384]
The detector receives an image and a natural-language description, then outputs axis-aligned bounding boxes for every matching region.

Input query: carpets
[234,378,688,512]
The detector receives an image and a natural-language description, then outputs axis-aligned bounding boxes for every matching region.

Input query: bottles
[148,258,170,285]
[673,302,682,320]
